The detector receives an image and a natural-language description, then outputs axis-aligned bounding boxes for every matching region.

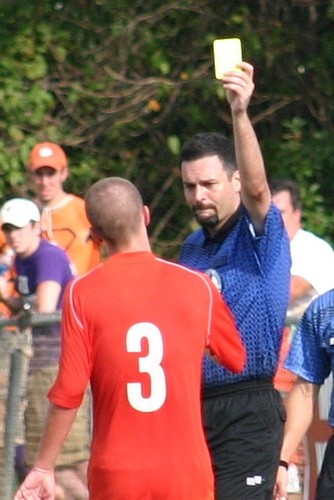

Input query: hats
[30,142,68,173]
[0,198,40,229]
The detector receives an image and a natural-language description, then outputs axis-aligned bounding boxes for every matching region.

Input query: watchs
[278,460,289,471]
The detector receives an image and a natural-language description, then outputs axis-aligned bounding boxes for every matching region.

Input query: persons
[178,61,293,500]
[0,200,90,500]
[266,180,334,500]
[271,288,334,500]
[0,143,101,276]
[12,177,248,500]
